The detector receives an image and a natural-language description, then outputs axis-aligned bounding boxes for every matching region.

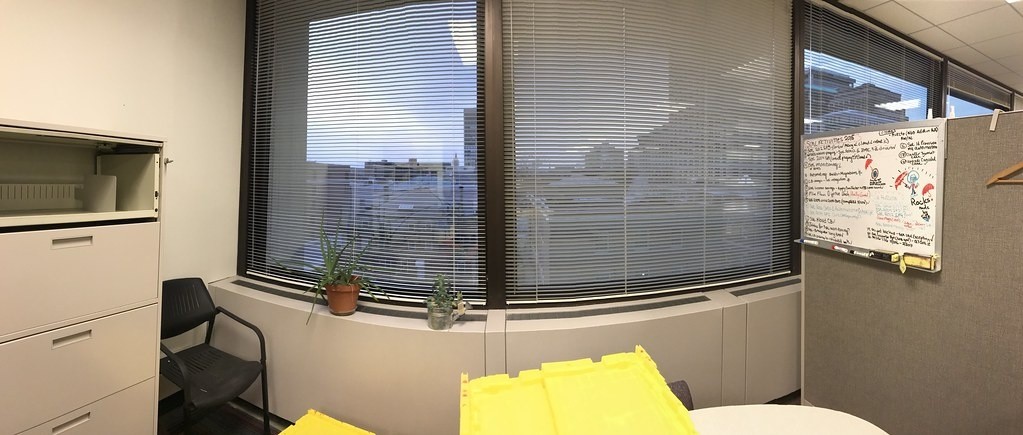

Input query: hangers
[987,161,1023,185]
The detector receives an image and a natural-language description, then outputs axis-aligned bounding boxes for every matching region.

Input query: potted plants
[269,207,388,325]
[423,274,468,331]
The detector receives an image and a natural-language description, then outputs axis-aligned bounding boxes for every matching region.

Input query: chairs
[162,277,271,435]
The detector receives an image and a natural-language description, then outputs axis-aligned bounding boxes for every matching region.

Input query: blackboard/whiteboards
[794,116,949,275]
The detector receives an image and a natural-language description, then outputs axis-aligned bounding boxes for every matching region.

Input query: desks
[688,404,891,435]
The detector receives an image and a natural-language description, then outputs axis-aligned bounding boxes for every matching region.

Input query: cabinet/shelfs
[0,119,166,435]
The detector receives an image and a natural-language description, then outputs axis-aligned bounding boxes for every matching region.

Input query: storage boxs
[459,343,702,435]
